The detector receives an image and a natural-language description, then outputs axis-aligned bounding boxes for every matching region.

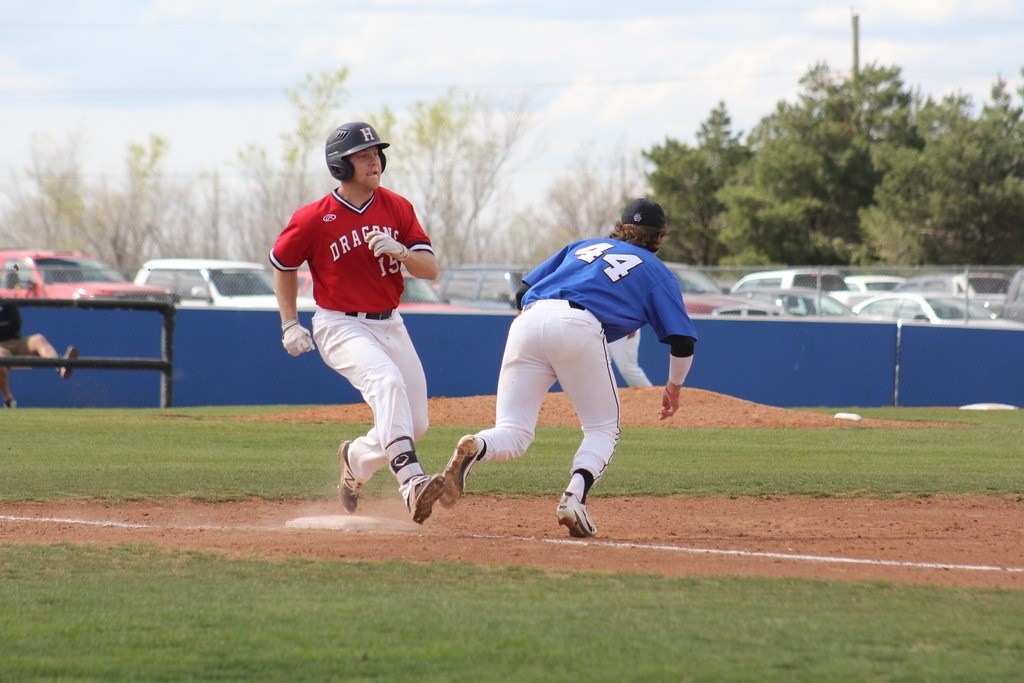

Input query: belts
[344,310,393,320]
[524,298,585,310]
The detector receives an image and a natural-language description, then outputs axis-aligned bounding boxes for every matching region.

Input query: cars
[0,250,183,307]
[296,271,477,312]
[133,259,313,311]
[661,259,1024,332]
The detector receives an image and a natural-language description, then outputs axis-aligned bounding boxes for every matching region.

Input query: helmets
[325,121,391,181]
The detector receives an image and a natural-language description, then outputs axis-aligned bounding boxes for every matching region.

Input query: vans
[438,264,534,312]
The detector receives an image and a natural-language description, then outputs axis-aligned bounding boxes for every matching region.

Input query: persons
[608,328,653,388]
[0,301,78,408]
[439,198,698,538]
[268,122,446,525]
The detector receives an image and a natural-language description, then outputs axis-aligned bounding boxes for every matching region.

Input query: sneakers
[556,491,597,537]
[338,439,361,514]
[406,473,446,524]
[438,434,478,508]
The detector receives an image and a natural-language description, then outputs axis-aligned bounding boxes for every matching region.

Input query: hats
[622,198,666,230]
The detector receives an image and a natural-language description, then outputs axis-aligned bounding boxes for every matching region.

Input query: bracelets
[665,387,679,399]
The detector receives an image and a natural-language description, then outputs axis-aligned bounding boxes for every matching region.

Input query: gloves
[365,230,409,261]
[281,317,315,356]
